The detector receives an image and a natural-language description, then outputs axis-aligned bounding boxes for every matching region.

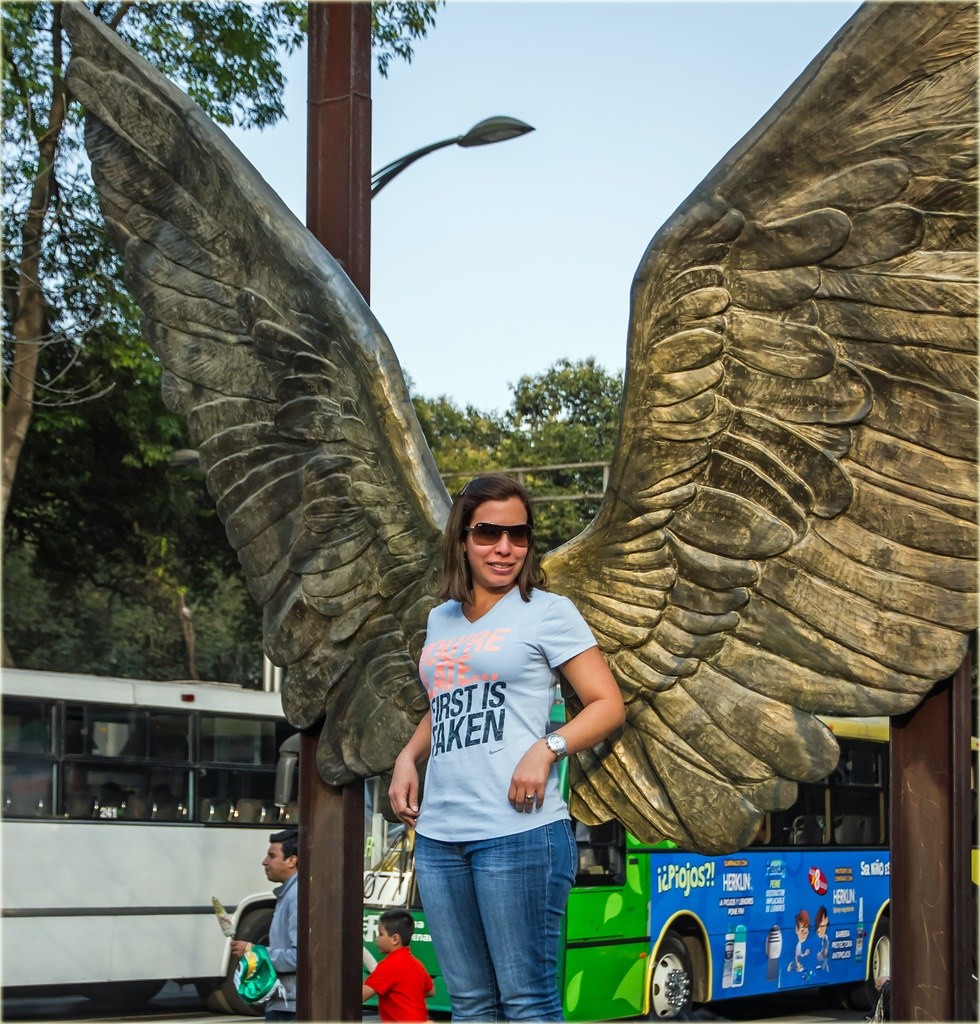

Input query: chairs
[208,799,235,820]
[790,816,823,844]
[277,801,298,823]
[63,793,99,817]
[152,798,182,818]
[233,797,265,822]
[833,815,868,844]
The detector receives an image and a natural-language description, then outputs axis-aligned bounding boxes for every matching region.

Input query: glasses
[465,522,534,547]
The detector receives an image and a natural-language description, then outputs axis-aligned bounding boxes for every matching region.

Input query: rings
[525,794,535,800]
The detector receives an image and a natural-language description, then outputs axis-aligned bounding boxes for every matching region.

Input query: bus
[355,711,980,1024]
[1,663,288,1014]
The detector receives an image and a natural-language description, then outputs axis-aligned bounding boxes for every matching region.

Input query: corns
[212,896,236,937]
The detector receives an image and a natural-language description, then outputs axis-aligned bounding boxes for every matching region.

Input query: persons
[388,477,626,1024]
[231,831,299,1023]
[362,911,432,1024]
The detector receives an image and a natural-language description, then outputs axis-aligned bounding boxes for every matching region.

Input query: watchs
[541,732,568,763]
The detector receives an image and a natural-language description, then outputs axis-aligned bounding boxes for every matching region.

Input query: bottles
[855,897,864,962]
[732,923,746,987]
[721,922,735,989]
